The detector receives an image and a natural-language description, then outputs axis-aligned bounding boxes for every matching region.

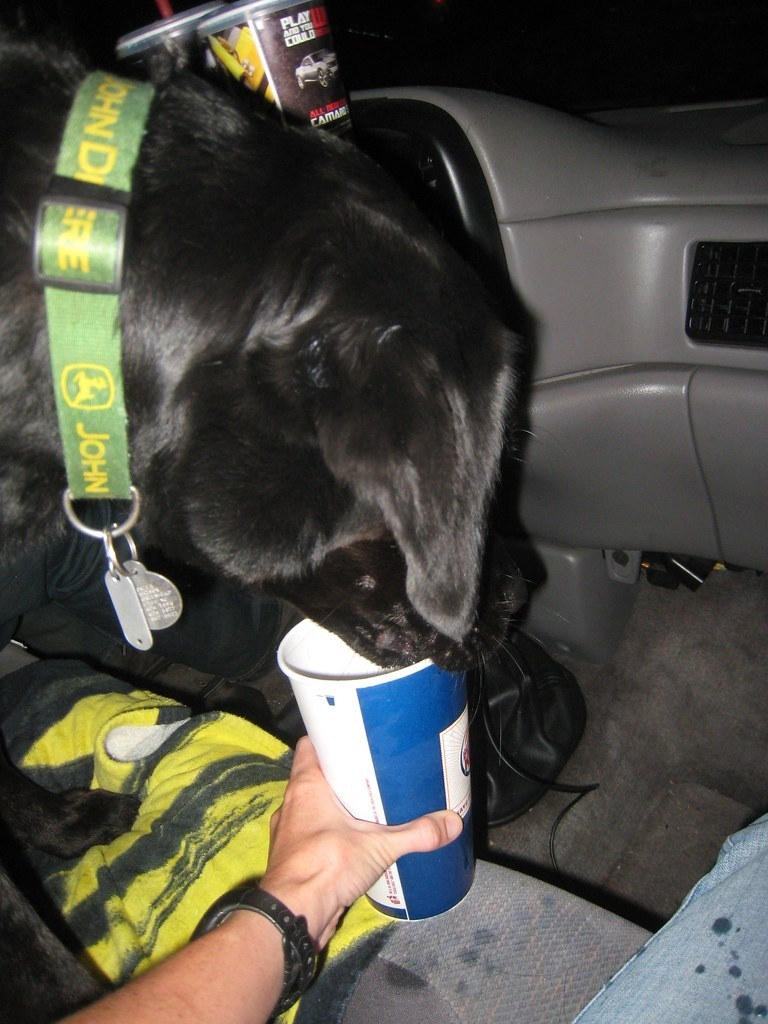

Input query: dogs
[1,35,536,862]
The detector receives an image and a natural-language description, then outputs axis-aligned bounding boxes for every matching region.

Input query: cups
[198,0,352,142]
[116,0,225,79]
[277,617,474,921]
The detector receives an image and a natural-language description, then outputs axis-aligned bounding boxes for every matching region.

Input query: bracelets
[189,882,316,1019]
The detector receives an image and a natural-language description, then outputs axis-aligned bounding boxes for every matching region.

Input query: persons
[55,736,768,1024]
